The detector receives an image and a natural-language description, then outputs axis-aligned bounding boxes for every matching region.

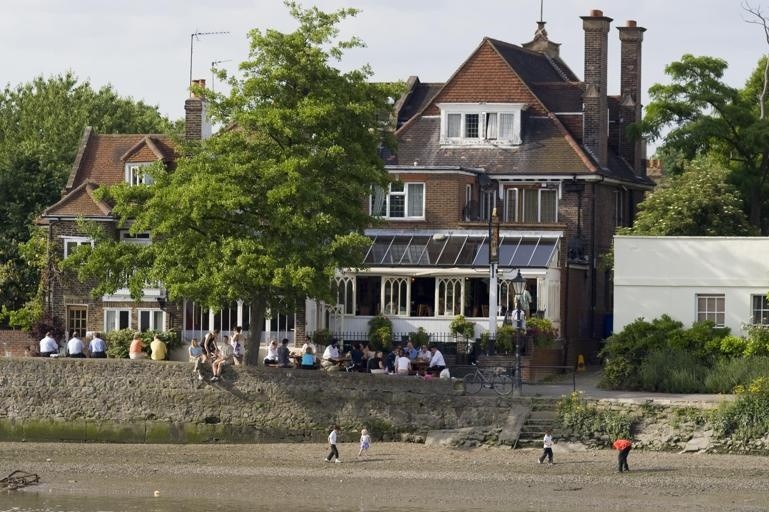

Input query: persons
[538,431,553,465]
[67,332,86,357]
[358,429,371,456]
[188,326,446,381]
[610,440,631,472]
[325,426,342,463]
[39,332,58,357]
[87,333,107,358]
[150,335,168,360]
[129,335,148,359]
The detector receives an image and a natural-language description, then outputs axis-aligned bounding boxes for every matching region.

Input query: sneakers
[324,458,342,464]
[197,376,222,382]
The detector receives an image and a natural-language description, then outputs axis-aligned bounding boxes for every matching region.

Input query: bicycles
[463,359,514,396]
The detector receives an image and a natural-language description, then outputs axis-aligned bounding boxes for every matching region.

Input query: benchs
[268,352,444,377]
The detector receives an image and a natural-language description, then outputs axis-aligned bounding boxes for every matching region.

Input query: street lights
[511,268,526,395]
[487,203,501,346]
